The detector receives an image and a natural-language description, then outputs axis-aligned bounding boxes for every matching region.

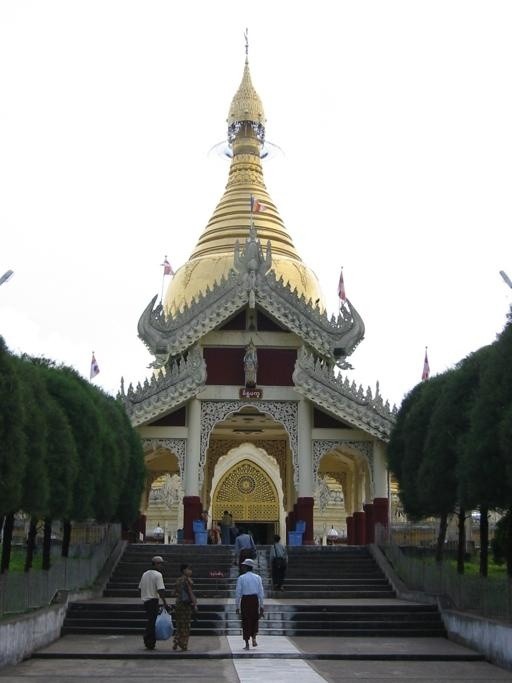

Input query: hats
[241,559,256,567]
[239,528,248,534]
[152,556,163,563]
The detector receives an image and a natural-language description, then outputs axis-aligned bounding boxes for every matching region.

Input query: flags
[164,259,177,278]
[90,355,100,379]
[338,271,349,300]
[422,351,430,380]
[251,196,267,212]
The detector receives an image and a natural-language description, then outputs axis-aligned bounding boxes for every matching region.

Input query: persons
[270,535,289,592]
[173,563,198,652]
[155,523,163,543]
[222,510,232,544]
[139,556,171,652]
[234,527,257,575]
[234,558,266,646]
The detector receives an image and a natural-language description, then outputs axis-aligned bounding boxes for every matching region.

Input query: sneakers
[173,646,177,649]
[243,643,257,650]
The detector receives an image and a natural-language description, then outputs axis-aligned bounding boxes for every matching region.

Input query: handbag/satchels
[182,589,192,603]
[272,557,286,569]
[240,549,256,559]
